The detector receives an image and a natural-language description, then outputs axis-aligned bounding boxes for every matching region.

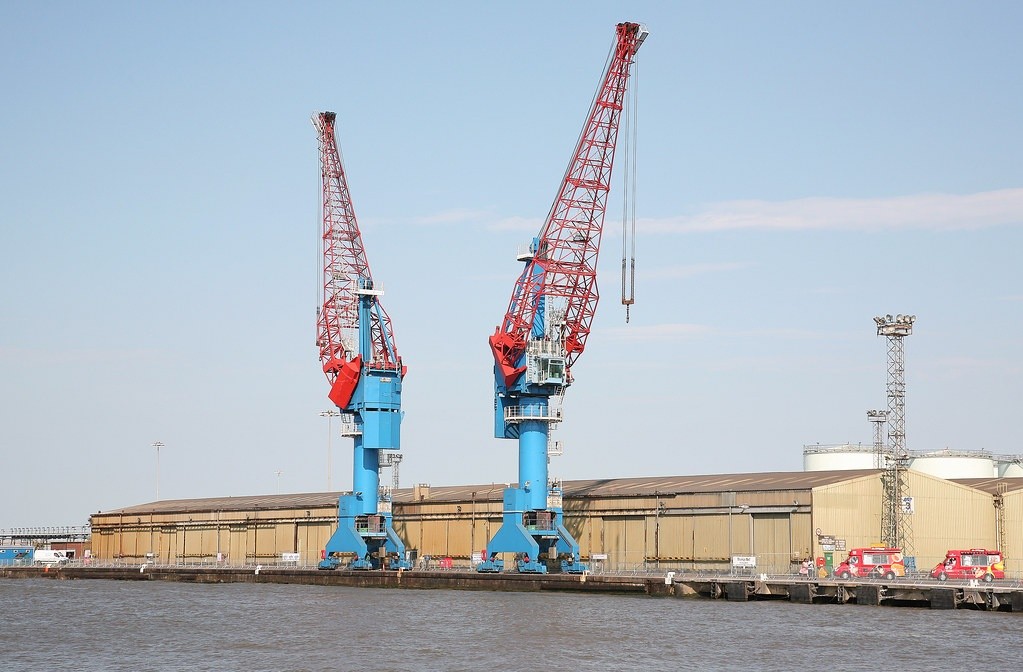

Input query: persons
[855,557,858,562]
[800,556,814,580]
[511,553,516,568]
[950,558,956,564]
[419,553,431,568]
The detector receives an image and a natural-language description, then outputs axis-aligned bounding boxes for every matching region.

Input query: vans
[930,549,1006,582]
[34,550,69,566]
[832,547,905,581]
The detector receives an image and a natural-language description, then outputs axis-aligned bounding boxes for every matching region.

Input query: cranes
[310,110,413,574]
[477,21,649,574]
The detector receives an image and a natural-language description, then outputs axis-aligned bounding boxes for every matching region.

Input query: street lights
[152,441,168,500]
[319,411,341,491]
